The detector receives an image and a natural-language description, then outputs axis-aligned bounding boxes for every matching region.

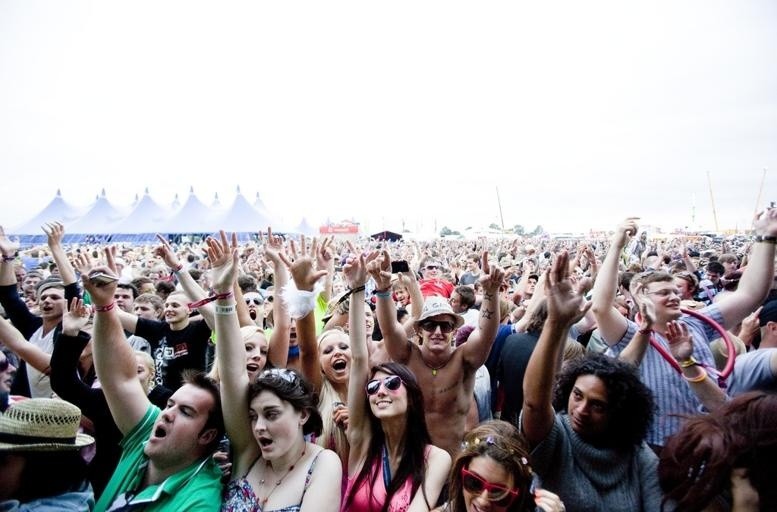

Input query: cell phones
[78,271,119,289]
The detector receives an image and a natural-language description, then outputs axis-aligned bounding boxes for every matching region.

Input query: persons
[0,205,777,512]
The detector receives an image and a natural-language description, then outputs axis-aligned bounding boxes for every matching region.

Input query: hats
[413,297,464,332]
[1,398,96,453]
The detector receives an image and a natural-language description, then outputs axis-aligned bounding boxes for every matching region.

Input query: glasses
[243,296,261,307]
[366,376,401,394]
[0,357,10,373]
[421,266,437,271]
[420,321,455,333]
[259,368,296,383]
[460,465,519,509]
